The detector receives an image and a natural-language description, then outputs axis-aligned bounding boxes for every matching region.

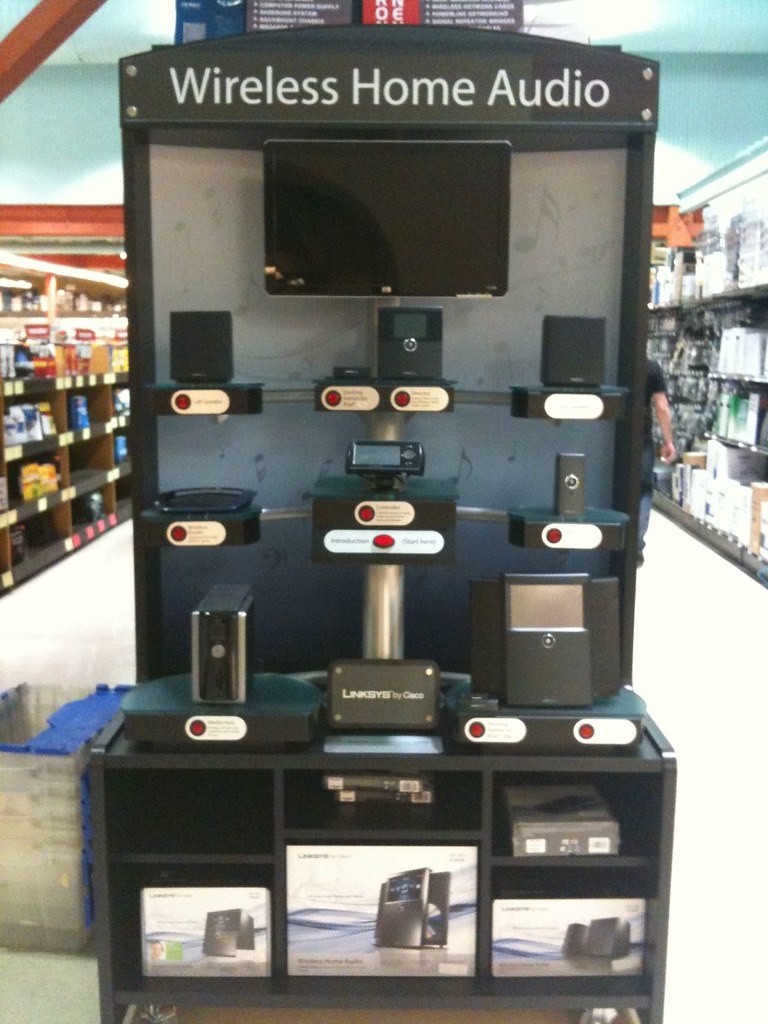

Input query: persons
[637,359,676,568]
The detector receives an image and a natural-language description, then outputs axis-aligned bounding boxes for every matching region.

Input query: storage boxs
[1,341,128,380]
[707,440,763,479]
[670,472,676,500]
[725,477,761,541]
[715,474,726,536]
[20,403,44,442]
[690,469,707,525]
[675,463,684,506]
[737,486,753,549]
[35,402,57,436]
[749,482,768,564]
[39,463,58,495]
[18,463,42,500]
[682,452,707,516]
[704,477,714,531]
[3,405,28,447]
[70,395,89,429]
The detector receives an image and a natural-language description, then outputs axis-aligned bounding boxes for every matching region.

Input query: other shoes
[635,553,644,567]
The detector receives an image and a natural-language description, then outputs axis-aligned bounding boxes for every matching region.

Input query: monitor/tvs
[261,138,511,298]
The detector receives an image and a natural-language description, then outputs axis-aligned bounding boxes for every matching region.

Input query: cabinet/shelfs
[0,250,132,595]
[644,209,768,591]
[89,711,677,1024]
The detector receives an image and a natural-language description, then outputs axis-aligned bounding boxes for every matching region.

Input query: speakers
[170,311,234,383]
[540,316,606,386]
